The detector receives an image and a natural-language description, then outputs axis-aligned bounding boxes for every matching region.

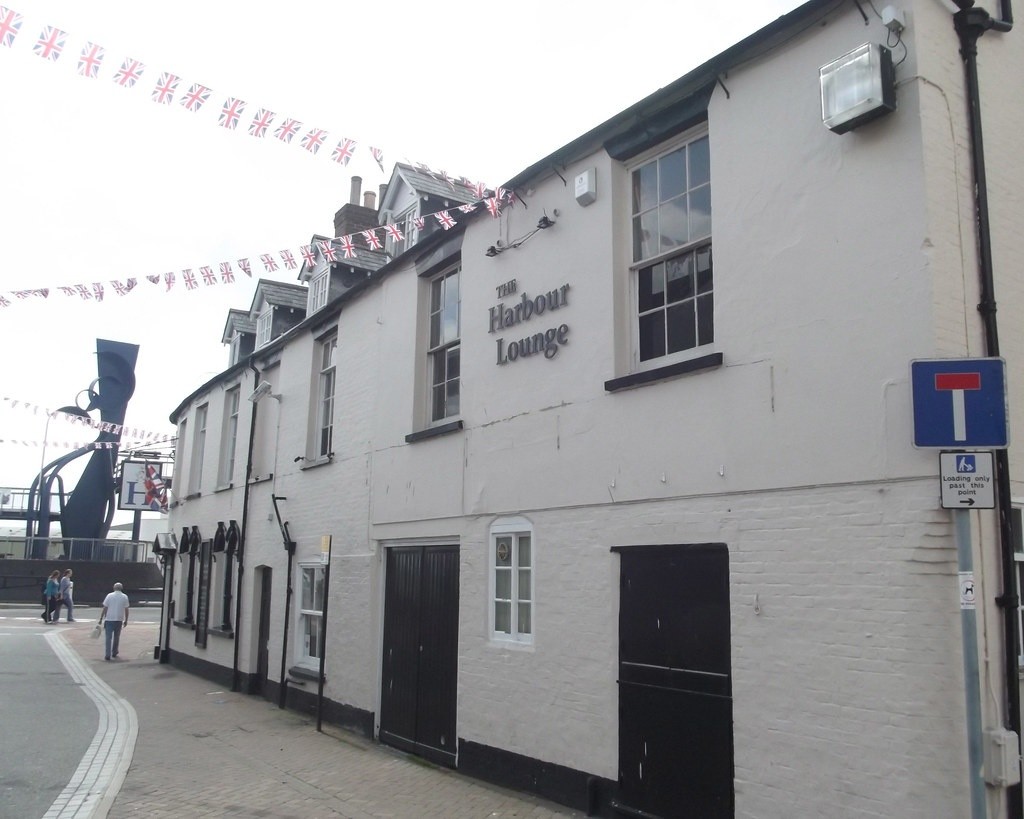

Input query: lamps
[536,216,555,230]
[485,246,501,258]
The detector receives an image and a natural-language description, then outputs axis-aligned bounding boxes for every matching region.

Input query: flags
[112,57,145,89]
[273,119,303,144]
[248,107,276,139]
[0,5,25,47]
[31,25,69,61]
[0,155,515,309]
[0,394,175,450]
[145,460,169,514]
[77,41,105,79]
[329,138,357,166]
[317,240,340,262]
[179,83,212,112]
[369,147,385,173]
[299,128,329,154]
[217,96,247,130]
[280,249,298,269]
[150,72,179,106]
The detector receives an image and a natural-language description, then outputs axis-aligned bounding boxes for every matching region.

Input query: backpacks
[40,582,47,605]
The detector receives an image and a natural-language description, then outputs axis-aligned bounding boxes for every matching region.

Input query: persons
[52,569,76,622]
[98,583,130,660]
[41,570,60,624]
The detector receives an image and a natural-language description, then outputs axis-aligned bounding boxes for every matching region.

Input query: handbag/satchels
[91,624,101,639]
[56,592,61,598]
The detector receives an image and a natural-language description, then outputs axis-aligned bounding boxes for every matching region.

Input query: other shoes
[41,613,46,622]
[68,619,74,622]
[55,619,58,624]
[112,650,119,657]
[105,654,110,660]
[47,619,54,624]
